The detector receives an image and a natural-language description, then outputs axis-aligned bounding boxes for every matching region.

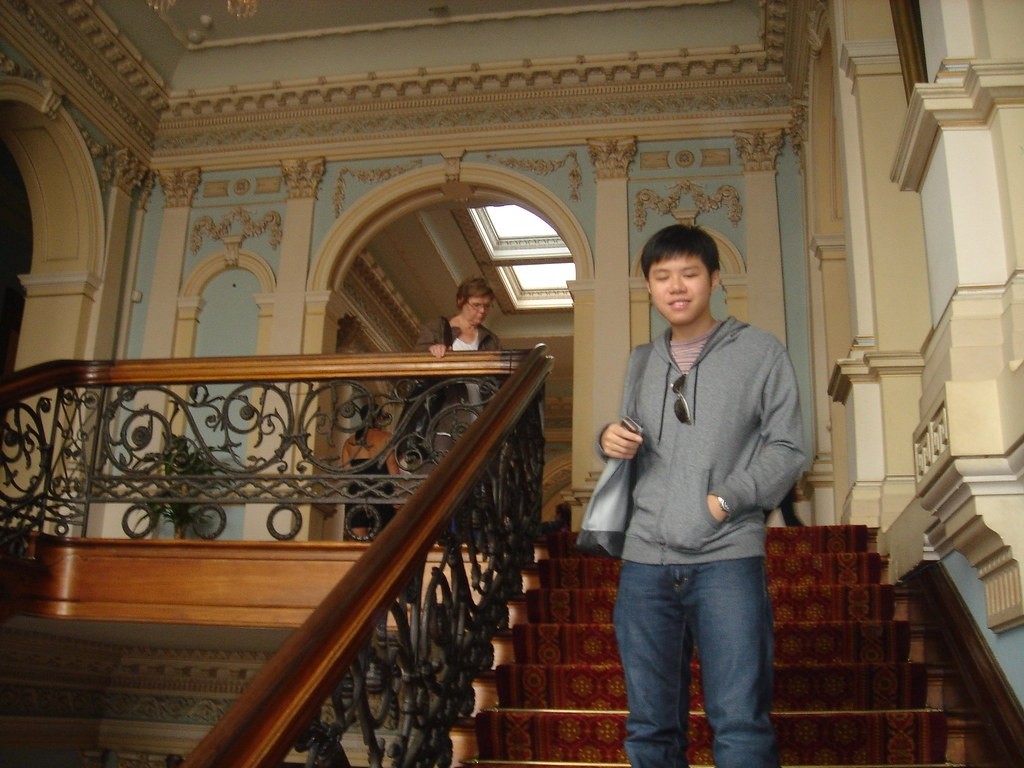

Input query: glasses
[671,373,693,426]
[466,298,492,313]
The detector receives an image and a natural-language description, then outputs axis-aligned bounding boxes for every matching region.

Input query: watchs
[717,496,730,513]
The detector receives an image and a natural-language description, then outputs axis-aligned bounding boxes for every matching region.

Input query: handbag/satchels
[575,338,653,559]
[391,378,469,465]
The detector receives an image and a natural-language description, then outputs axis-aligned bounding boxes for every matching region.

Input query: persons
[411,276,504,549]
[595,225,806,768]
[342,403,401,542]
